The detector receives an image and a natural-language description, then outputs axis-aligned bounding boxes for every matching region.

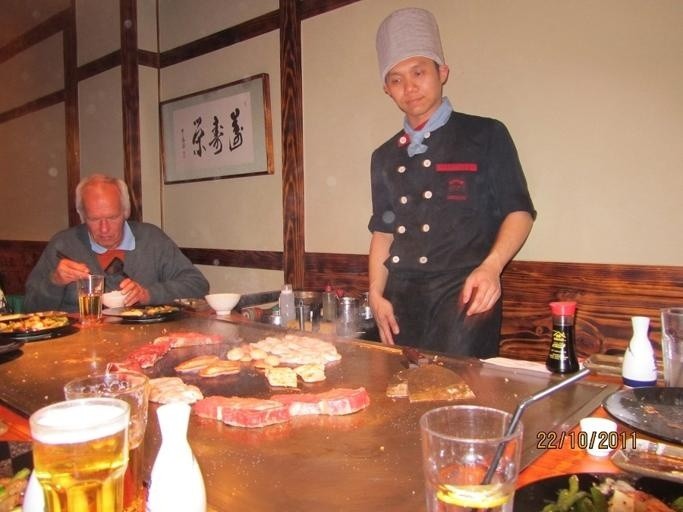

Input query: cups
[660,307,683,388]
[420,405,523,512]
[75,275,105,325]
[28,371,206,512]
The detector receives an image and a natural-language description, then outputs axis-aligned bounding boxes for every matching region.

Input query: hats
[376,7,445,86]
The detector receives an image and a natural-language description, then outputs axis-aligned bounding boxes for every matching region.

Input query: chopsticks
[57,250,94,275]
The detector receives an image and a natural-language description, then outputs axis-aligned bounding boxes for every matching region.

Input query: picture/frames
[158,73,275,184]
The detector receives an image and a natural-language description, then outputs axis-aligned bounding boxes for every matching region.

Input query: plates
[0,309,78,358]
[513,387,682,512]
[101,305,185,322]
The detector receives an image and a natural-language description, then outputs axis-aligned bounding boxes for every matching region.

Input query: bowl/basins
[104,291,129,308]
[205,293,242,315]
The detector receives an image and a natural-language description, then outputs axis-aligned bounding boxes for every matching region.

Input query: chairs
[0,241,46,311]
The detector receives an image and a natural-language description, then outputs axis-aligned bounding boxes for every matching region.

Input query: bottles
[270,283,373,338]
[546,302,579,373]
[621,316,657,389]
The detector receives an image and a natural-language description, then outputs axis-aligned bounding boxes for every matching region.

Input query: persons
[367,57,538,361]
[24,173,211,313]
